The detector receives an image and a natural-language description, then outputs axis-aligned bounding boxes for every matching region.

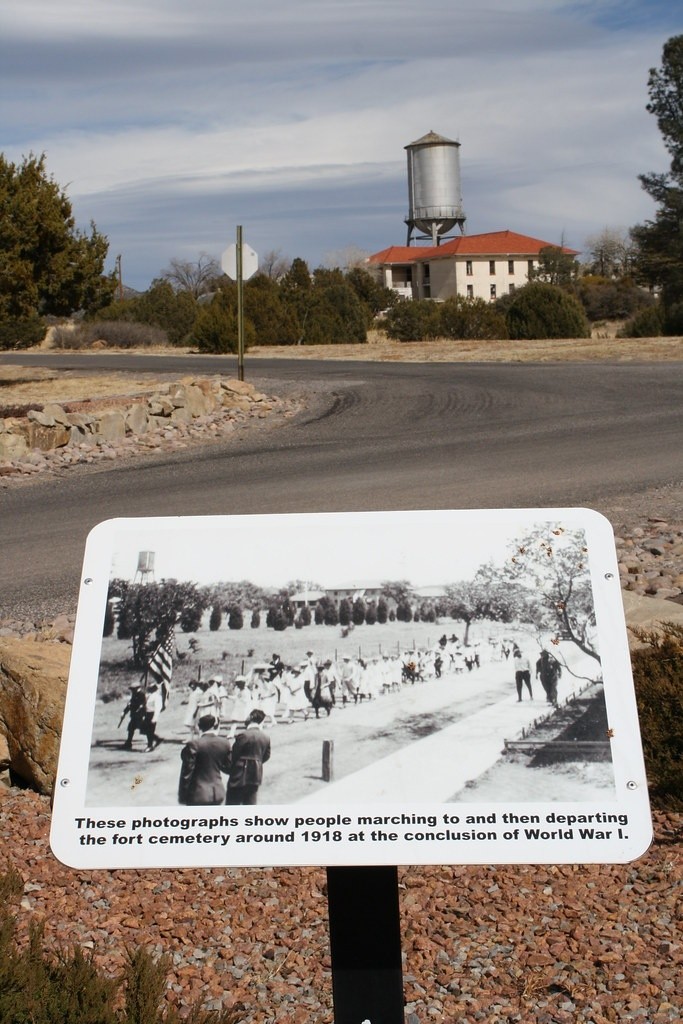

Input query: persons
[118,634,562,806]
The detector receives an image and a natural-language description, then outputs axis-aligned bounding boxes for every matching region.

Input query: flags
[145,627,174,712]
